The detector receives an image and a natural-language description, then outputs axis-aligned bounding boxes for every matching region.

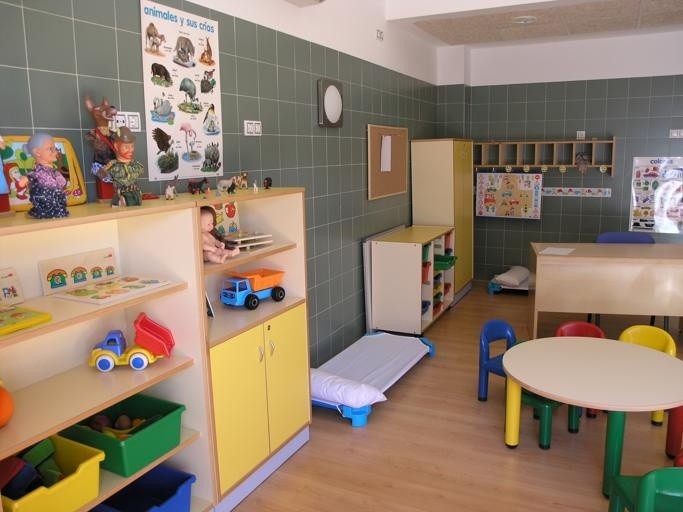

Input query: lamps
[316,75,344,131]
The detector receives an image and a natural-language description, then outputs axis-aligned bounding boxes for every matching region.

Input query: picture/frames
[365,123,409,203]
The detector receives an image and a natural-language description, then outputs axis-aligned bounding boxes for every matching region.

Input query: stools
[518,388,585,446]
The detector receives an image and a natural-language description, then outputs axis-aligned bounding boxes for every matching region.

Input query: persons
[19,133,68,218]
[103,125,145,207]
[200,206,240,264]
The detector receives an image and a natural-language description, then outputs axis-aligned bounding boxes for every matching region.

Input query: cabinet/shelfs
[0,186,225,512]
[406,134,476,304]
[367,221,453,335]
[179,175,313,508]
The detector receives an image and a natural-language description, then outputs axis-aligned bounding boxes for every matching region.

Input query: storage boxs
[70,392,183,476]
[94,463,197,512]
[1,434,105,512]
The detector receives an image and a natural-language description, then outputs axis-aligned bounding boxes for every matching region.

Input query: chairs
[603,465,681,511]
[611,324,675,428]
[584,229,672,331]
[472,317,515,403]
[543,317,606,420]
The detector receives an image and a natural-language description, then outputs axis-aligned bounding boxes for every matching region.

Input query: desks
[494,337,681,500]
[521,238,681,345]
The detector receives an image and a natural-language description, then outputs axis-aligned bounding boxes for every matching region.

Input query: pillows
[492,264,529,287]
[306,365,387,409]
